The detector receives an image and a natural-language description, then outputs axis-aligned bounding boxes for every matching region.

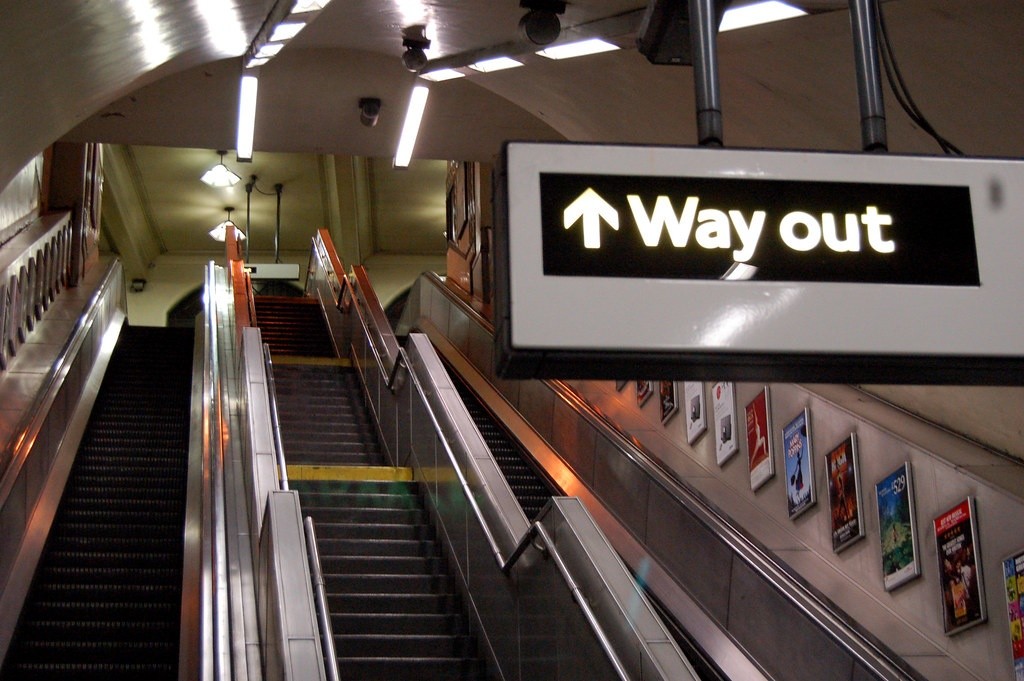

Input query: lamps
[200,151,242,188]
[235,0,332,162]
[208,207,246,242]
[131,279,147,292]
[392,0,810,171]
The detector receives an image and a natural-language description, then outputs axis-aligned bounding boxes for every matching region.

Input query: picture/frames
[683,381,707,445]
[744,386,775,492]
[448,161,476,245]
[932,496,988,635]
[825,431,865,553]
[659,381,679,426]
[1001,549,1024,681]
[86,142,101,229]
[782,406,817,520]
[616,380,628,392]
[875,460,921,591]
[0,220,73,371]
[711,381,738,467]
[637,380,653,407]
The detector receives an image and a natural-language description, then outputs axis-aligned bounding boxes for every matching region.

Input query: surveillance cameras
[634,0,731,66]
